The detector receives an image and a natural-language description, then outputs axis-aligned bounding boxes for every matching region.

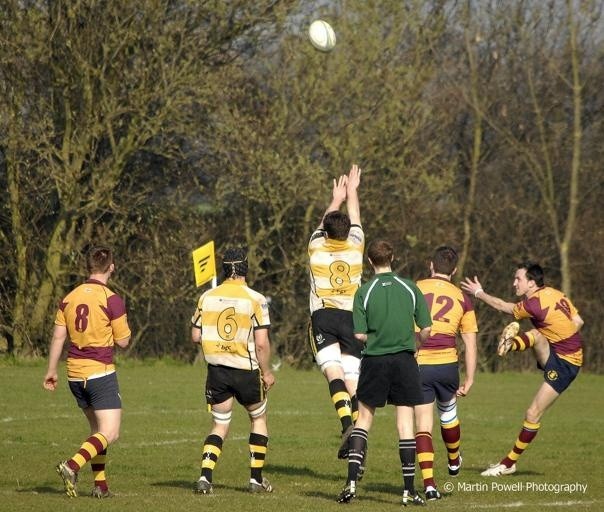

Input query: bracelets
[475,289,483,297]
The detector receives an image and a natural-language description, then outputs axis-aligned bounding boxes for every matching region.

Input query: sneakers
[195,477,211,493]
[448,452,463,476]
[91,485,115,497]
[425,487,441,501]
[55,461,78,498]
[497,321,519,356]
[480,462,515,477]
[402,489,426,507]
[339,426,353,459]
[337,480,357,502]
[248,478,273,494]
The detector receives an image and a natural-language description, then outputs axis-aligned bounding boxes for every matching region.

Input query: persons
[191,248,277,494]
[336,238,427,506]
[412,246,479,502]
[307,163,365,458]
[43,246,131,498]
[460,260,585,476]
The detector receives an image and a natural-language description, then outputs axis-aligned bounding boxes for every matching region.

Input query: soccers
[308,19,337,51]
